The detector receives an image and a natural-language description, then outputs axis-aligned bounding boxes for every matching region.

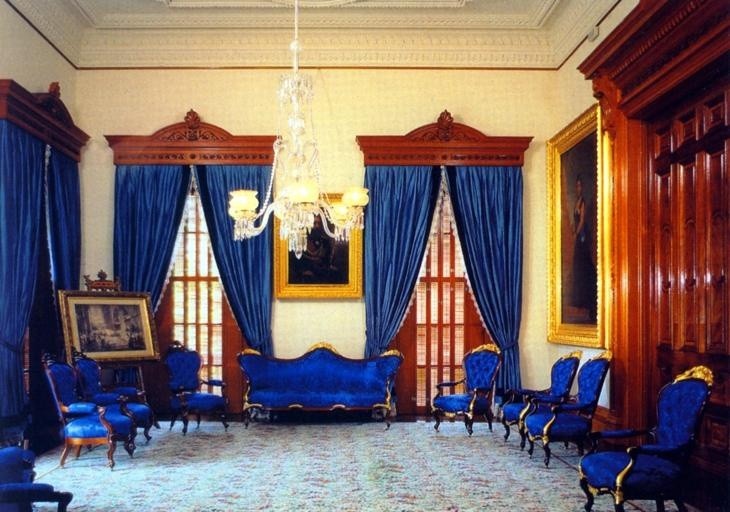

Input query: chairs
[0,341,229,512]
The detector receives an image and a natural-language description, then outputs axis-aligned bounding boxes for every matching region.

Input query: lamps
[226,1,369,259]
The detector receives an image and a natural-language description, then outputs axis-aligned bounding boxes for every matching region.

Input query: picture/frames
[545,102,605,349]
[272,192,364,300]
[57,289,162,365]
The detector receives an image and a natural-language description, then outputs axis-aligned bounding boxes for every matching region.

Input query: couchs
[235,342,405,428]
